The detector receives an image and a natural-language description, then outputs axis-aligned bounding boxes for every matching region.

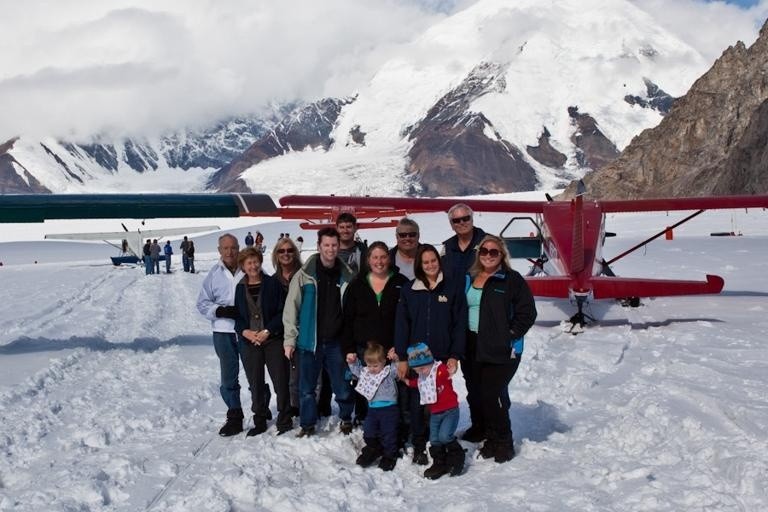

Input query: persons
[439,204,492,443]
[144,236,161,275]
[196,234,273,437]
[282,227,353,437]
[164,240,173,274]
[279,233,289,240]
[318,213,368,427]
[120,238,129,256]
[458,236,537,464]
[234,247,294,436]
[180,236,195,274]
[335,241,410,366]
[393,243,467,466]
[387,218,421,284]
[398,342,465,479]
[345,342,400,473]
[272,237,321,415]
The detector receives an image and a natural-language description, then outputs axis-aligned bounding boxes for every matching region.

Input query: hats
[407,342,434,368]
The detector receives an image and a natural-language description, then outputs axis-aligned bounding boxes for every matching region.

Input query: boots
[355,432,466,480]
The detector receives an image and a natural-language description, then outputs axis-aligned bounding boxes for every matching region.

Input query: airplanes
[42,223,221,266]
[240,208,409,229]
[237,176,767,337]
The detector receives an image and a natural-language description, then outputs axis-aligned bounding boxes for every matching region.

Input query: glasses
[480,247,502,258]
[278,248,294,253]
[398,232,418,237]
[452,215,471,223]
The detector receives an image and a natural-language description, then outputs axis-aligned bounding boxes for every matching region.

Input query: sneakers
[462,421,515,463]
[219,401,367,439]
